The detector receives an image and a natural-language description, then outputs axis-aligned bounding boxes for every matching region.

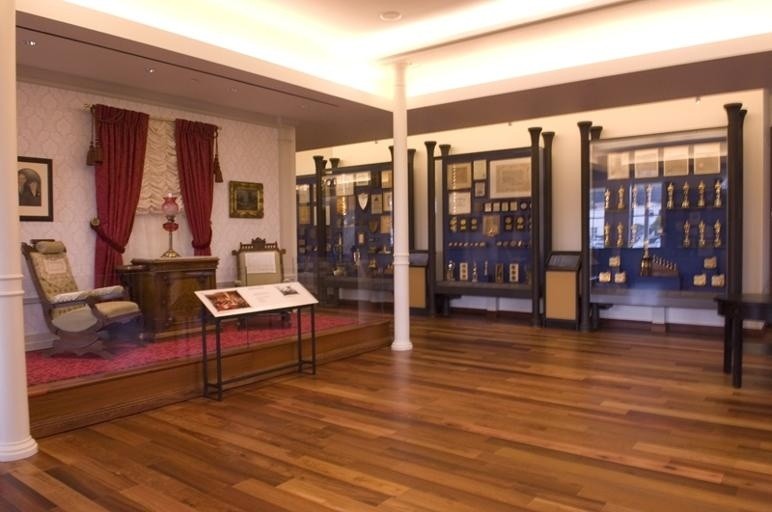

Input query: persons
[18,170,36,206]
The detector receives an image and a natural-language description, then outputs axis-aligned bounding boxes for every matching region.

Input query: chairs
[21,238,142,355]
[232,237,292,329]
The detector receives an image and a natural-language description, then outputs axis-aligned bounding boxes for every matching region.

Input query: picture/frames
[228,180,264,219]
[17,155,54,222]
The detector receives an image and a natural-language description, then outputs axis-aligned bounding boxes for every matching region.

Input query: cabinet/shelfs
[201,303,315,401]
[130,255,223,344]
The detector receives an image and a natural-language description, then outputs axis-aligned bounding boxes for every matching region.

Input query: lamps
[162,192,182,258]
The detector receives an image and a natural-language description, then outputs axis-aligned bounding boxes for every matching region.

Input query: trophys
[471,261,479,283]
[446,260,456,282]
[639,239,653,276]
[602,177,721,248]
[333,218,394,280]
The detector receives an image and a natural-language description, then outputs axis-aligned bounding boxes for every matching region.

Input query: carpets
[26,312,359,387]
